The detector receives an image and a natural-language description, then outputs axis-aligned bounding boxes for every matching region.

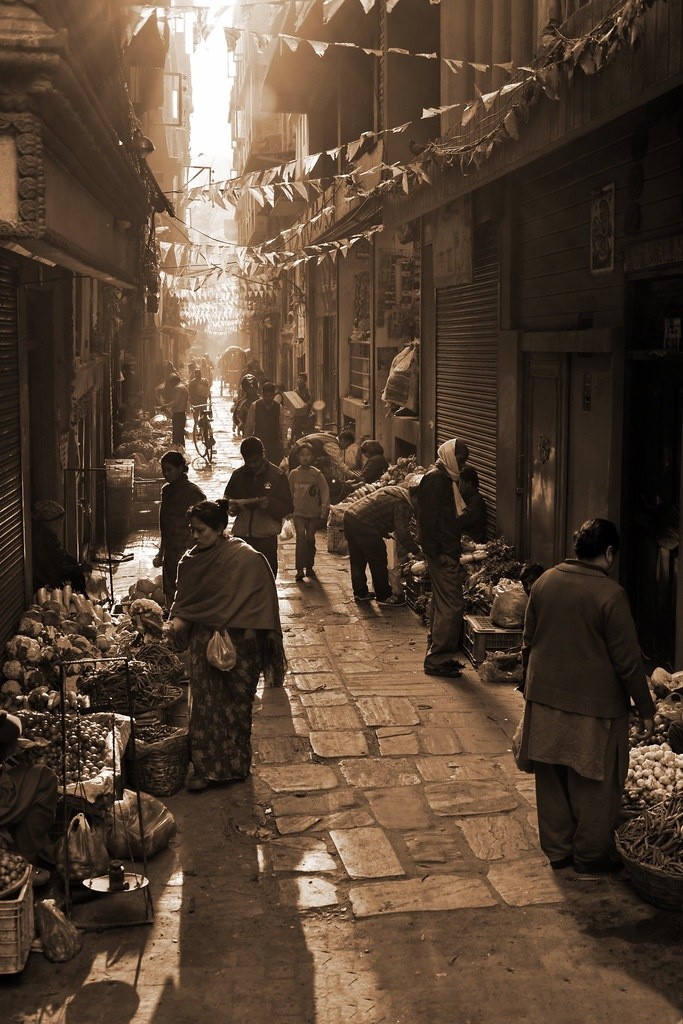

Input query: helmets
[241,374,258,392]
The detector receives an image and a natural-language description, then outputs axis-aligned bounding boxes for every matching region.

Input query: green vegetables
[124,724,192,796]
[406,536,526,623]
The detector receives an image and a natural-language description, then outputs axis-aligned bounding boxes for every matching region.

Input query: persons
[343,439,487,678]
[223,436,292,580]
[121,364,140,419]
[288,429,359,480]
[517,518,658,874]
[162,353,216,448]
[153,450,208,612]
[288,443,331,578]
[336,439,389,504]
[0,710,59,887]
[164,499,289,792]
[230,360,314,467]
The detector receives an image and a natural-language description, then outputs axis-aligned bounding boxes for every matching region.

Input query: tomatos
[11,710,109,783]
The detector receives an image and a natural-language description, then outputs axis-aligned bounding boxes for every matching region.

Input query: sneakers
[354,591,374,601]
[376,594,406,606]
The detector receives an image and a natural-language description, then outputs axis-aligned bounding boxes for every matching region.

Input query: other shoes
[306,569,315,577]
[573,856,625,874]
[295,571,304,579]
[549,856,571,870]
[425,666,462,678]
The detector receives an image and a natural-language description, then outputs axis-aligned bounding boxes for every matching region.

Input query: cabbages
[23,600,110,648]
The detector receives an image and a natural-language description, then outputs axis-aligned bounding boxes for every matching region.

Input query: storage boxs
[406,574,431,611]
[282,391,305,416]
[104,458,135,489]
[0,864,35,974]
[463,614,524,665]
[326,513,344,552]
[110,604,125,618]
[131,501,162,525]
[133,478,166,501]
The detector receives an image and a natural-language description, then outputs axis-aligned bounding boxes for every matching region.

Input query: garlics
[621,742,683,810]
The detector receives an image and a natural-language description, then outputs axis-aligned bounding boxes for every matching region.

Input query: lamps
[127,128,155,155]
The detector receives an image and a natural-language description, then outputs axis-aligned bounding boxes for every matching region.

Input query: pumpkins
[651,667,683,699]
[128,575,167,606]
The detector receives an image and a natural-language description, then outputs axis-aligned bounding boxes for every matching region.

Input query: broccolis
[129,598,164,616]
[381,457,424,485]
[0,618,102,696]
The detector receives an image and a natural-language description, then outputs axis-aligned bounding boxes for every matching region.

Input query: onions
[0,848,27,891]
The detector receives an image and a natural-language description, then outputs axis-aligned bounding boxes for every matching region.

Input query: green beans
[79,641,185,713]
[616,788,683,876]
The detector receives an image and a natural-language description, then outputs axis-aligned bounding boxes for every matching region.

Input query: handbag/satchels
[108,788,174,858]
[52,813,110,882]
[206,630,236,672]
[35,899,83,962]
